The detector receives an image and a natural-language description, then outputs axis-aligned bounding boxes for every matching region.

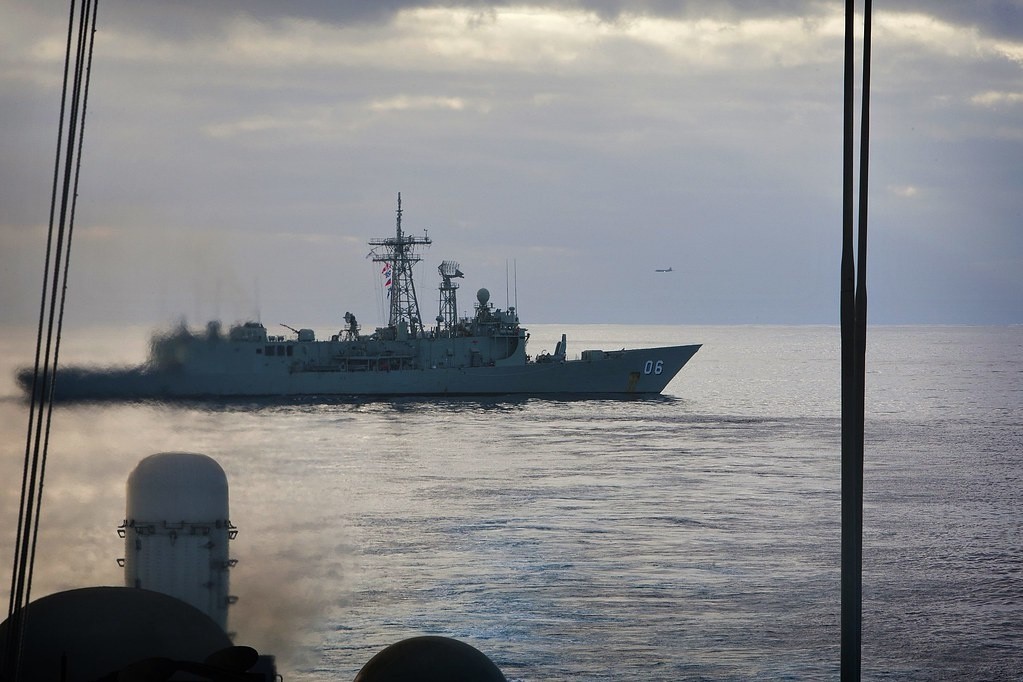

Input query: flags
[382,263,391,286]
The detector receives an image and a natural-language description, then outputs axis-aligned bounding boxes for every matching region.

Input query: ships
[16,191,705,405]
